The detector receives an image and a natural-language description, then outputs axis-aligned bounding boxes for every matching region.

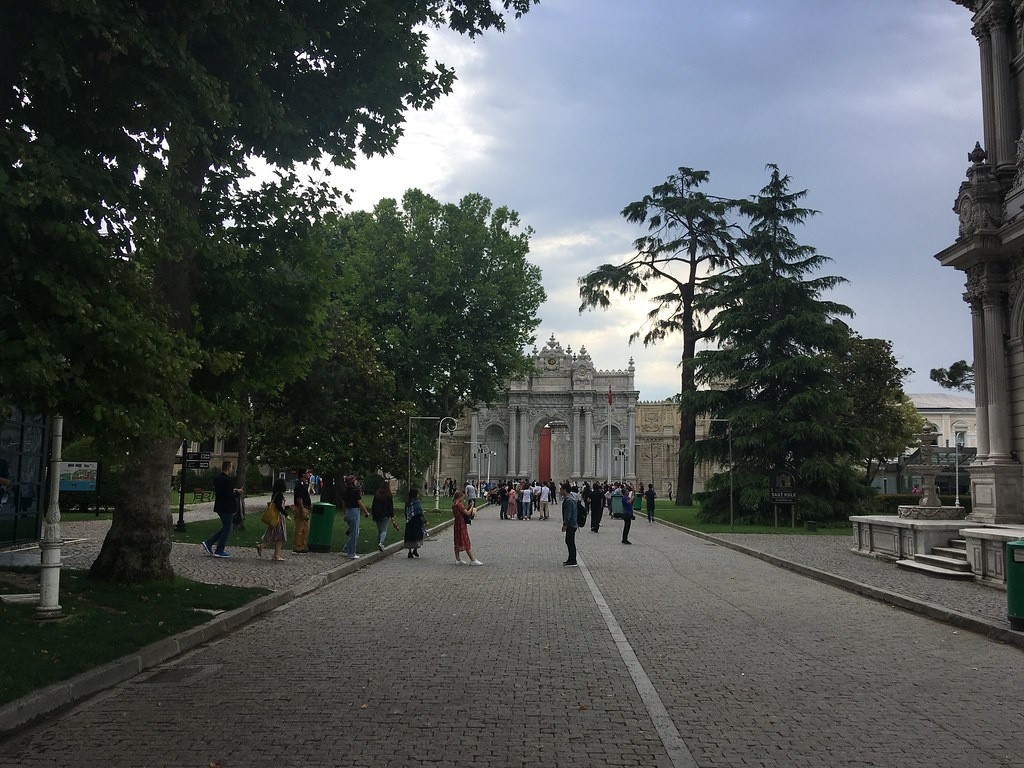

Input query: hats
[343,475,356,483]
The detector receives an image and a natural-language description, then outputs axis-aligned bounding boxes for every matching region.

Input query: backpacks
[565,494,587,527]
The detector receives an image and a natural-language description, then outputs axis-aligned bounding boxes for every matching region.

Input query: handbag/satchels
[604,491,611,499]
[260,493,284,527]
[456,503,471,525]
[466,502,474,520]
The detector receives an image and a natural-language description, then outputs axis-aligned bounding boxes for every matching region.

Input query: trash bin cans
[611,495,624,518]
[1005,541,1024,632]
[633,492,642,510]
[308,502,336,552]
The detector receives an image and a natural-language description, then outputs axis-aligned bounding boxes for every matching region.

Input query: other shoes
[256,544,263,557]
[563,558,577,567]
[338,552,348,557]
[347,553,359,559]
[622,540,631,545]
[591,527,598,533]
[470,559,483,566]
[502,515,549,521]
[408,553,414,558]
[455,558,466,565]
[272,556,286,561]
[378,543,385,552]
[291,549,308,555]
[412,550,419,557]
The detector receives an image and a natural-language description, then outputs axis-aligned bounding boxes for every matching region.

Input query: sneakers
[214,551,231,558]
[202,540,213,554]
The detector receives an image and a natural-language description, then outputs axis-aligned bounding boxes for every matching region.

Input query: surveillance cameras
[494,452,497,456]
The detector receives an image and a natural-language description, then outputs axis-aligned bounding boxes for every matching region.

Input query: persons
[668,482,672,501]
[342,475,370,558]
[202,461,243,558]
[480,477,557,520]
[912,485,921,493]
[644,484,657,522]
[621,488,636,544]
[590,483,604,533]
[464,482,478,508]
[425,483,428,496]
[404,488,429,559]
[472,481,475,487]
[292,468,311,555]
[638,483,644,493]
[452,492,483,566]
[558,479,635,515]
[257,478,292,561]
[443,478,456,497]
[308,473,320,495]
[371,481,395,551]
[561,483,578,567]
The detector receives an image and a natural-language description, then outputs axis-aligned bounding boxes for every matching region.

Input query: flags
[608,385,613,405]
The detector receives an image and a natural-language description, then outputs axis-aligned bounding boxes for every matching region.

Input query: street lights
[612,447,631,484]
[484,450,497,497]
[432,417,459,513]
[406,417,440,493]
[25,353,71,623]
[954,431,966,508]
[474,444,490,500]
